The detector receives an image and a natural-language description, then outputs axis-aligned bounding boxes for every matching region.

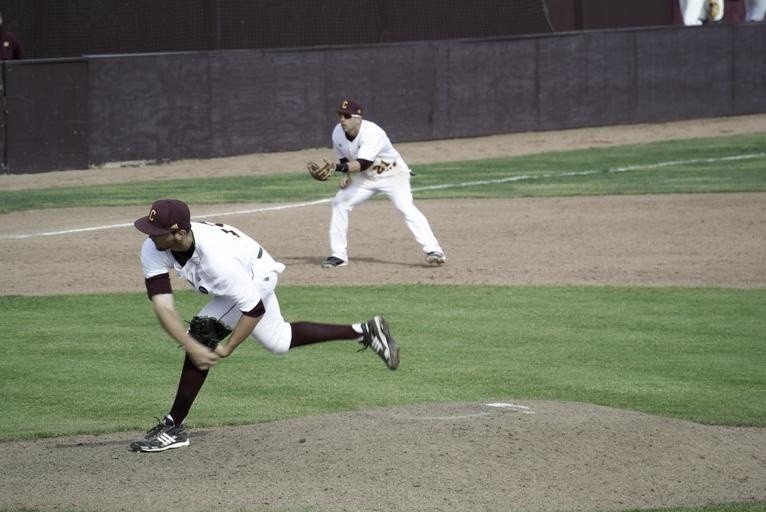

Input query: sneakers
[426,250,447,264]
[361,314,400,370]
[131,417,190,451]
[322,256,346,267]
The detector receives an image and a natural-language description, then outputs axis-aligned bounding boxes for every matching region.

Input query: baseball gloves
[187,316,231,351]
[308,159,335,181]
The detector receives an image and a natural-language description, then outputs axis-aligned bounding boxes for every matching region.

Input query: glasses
[344,114,361,119]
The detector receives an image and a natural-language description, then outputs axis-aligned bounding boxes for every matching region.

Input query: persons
[743,1,766,24]
[678,0,728,27]
[308,99,446,270]
[127,199,401,454]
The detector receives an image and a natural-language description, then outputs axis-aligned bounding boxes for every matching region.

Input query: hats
[134,199,189,235]
[334,100,363,115]
